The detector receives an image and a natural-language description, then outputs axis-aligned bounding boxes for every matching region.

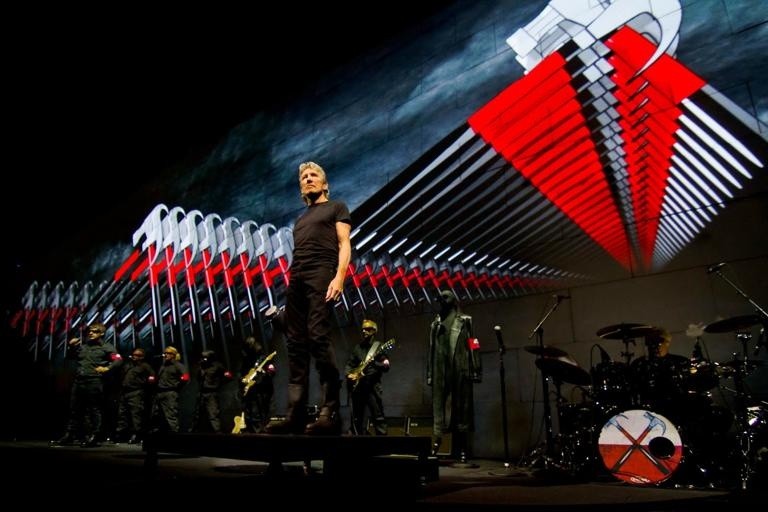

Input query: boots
[266,380,311,433]
[307,379,342,433]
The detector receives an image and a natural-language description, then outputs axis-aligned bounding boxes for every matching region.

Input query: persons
[266,162,353,433]
[426,289,482,462]
[49,319,391,449]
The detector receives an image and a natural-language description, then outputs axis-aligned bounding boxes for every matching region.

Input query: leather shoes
[127,435,141,444]
[107,432,123,441]
[80,436,98,448]
[50,437,72,445]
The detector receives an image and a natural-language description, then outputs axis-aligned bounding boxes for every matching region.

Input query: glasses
[89,329,100,335]
[163,351,174,355]
[361,327,376,331]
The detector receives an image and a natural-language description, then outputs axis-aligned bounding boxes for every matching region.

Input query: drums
[557,356,735,487]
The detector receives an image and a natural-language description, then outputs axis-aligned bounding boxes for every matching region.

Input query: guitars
[347,338,397,392]
[240,350,277,397]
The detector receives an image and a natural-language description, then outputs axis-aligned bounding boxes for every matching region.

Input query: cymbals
[523,344,570,357]
[702,313,767,334]
[596,323,671,340]
[534,357,592,386]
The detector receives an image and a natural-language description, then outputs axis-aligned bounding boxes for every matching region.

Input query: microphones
[553,295,572,300]
[68,336,83,349]
[705,262,726,274]
